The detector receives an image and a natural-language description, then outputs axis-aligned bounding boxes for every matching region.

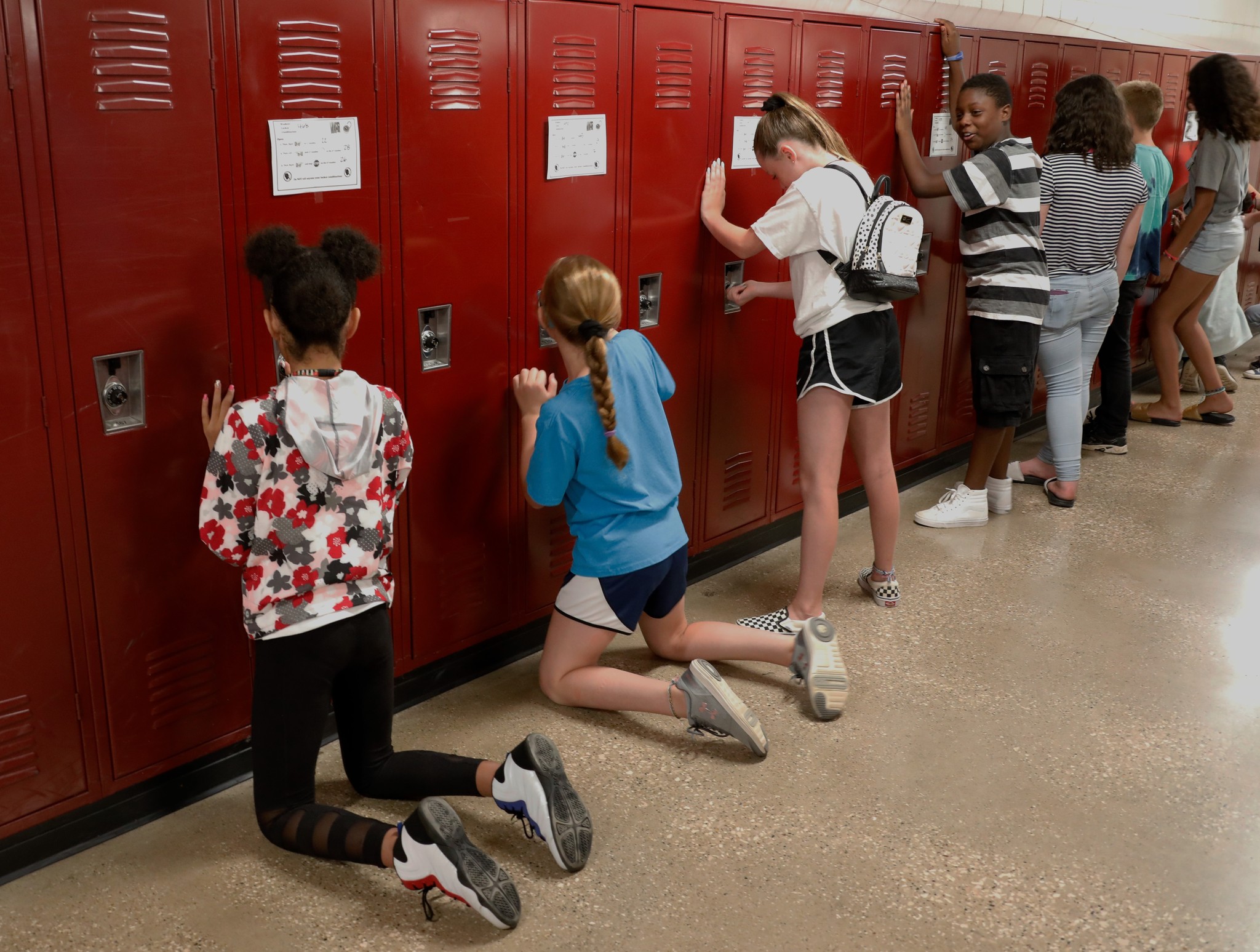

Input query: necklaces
[284,368,342,378]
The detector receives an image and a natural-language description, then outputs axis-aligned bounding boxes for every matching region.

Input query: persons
[1128,53,1260,427]
[1082,79,1174,455]
[198,227,592,930]
[514,254,850,759]
[895,18,1051,529]
[1167,181,1260,392]
[1243,304,1260,380]
[1005,74,1149,508]
[701,90,901,636]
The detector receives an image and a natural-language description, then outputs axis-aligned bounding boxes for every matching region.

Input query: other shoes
[1179,358,1201,392]
[858,567,900,607]
[1249,358,1260,369]
[1214,354,1238,392]
[734,606,827,637]
[1242,368,1260,380]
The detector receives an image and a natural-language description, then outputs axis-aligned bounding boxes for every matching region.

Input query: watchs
[946,51,963,61]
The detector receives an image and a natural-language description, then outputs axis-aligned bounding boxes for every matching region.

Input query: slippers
[1182,403,1236,424]
[1044,476,1074,508]
[1006,461,1046,485]
[1128,403,1181,426]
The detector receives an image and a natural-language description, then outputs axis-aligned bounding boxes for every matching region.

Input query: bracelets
[1163,250,1179,261]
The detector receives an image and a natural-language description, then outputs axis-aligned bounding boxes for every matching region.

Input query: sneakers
[787,616,851,722]
[491,732,593,874]
[914,483,989,528]
[952,474,1013,514]
[1081,410,1128,454]
[391,797,522,931]
[674,657,769,761]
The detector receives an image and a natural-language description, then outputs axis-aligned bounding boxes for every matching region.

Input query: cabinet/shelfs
[0,1,1260,883]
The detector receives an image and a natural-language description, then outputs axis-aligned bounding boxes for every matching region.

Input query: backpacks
[816,164,925,303]
